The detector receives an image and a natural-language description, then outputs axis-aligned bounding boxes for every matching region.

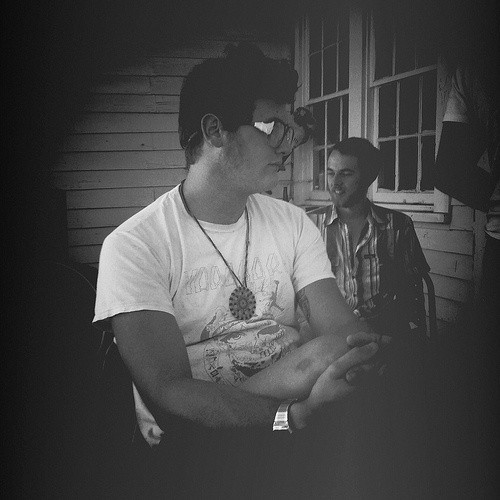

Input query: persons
[93,39,390,472]
[307,137,426,337]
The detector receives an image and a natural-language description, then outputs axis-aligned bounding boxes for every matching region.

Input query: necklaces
[181,180,257,320]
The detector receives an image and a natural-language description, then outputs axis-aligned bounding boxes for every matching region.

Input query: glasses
[235,119,295,150]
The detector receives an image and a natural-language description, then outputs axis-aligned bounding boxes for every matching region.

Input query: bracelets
[272,398,299,441]
